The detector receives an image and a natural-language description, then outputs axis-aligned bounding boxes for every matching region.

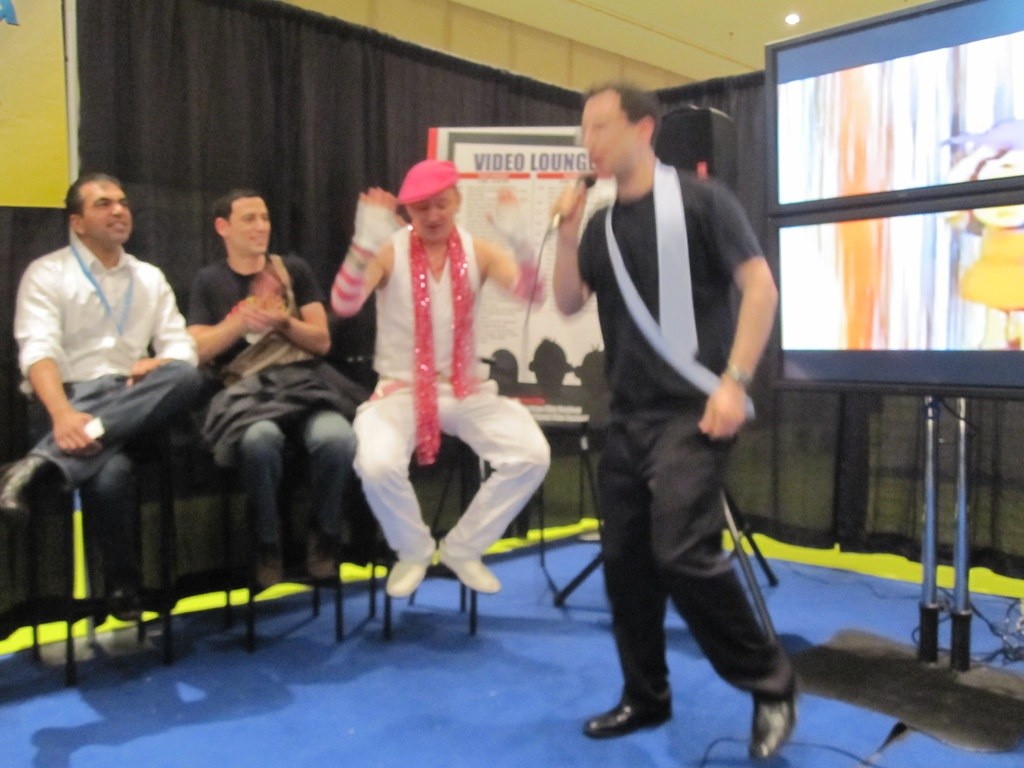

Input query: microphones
[550,172,597,233]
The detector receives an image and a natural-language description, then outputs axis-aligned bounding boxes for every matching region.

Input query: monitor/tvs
[767,187,1024,399]
[765,0,1024,216]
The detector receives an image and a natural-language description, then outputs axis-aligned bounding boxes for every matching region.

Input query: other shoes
[107,589,139,622]
[257,557,284,587]
[0,455,57,521]
[299,535,340,588]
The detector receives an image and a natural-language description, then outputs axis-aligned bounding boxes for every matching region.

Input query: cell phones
[84,414,107,439]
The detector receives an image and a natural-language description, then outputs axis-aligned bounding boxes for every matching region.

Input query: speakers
[652,102,739,196]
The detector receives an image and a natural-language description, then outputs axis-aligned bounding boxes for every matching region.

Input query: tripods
[550,473,783,651]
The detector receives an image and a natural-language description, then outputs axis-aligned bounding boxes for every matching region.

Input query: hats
[396,159,459,204]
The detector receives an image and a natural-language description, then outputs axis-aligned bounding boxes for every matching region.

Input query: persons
[188,187,361,590]
[552,80,800,761]
[328,160,553,599]
[1,168,199,616]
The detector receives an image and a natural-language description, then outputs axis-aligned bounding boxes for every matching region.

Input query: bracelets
[725,366,754,390]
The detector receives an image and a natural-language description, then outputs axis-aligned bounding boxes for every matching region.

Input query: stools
[31,422,778,689]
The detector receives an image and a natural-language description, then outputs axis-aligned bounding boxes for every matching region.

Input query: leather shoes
[386,550,433,597]
[583,698,672,740]
[748,695,797,762]
[438,540,502,594]
